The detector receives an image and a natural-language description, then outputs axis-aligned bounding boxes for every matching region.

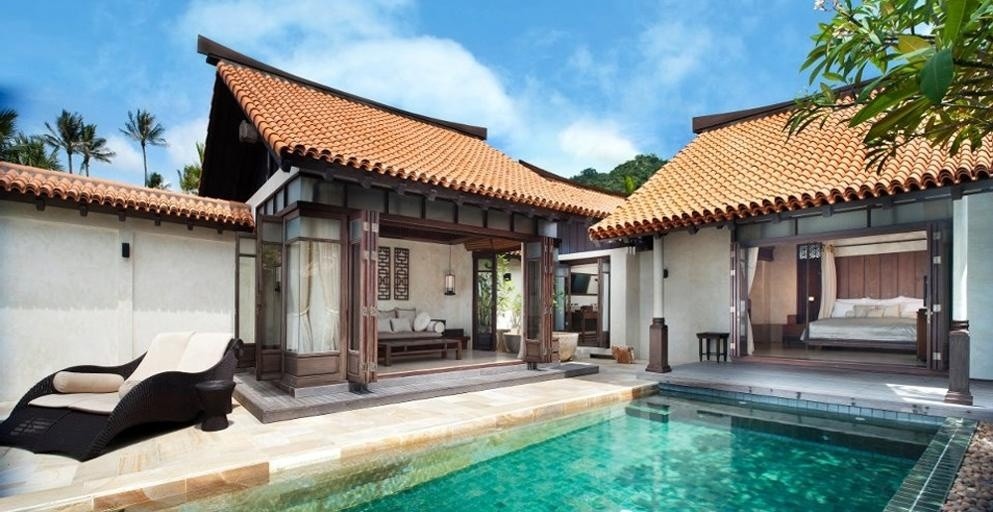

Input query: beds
[803,296,924,352]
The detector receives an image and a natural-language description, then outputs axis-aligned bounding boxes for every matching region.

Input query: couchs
[377,305,447,357]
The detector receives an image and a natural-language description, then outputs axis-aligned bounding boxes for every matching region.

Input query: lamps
[442,245,456,296]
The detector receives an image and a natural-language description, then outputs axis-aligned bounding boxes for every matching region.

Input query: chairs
[0,329,246,462]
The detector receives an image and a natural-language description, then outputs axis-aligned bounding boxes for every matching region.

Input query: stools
[695,331,730,363]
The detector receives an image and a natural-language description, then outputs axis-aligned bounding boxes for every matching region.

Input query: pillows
[377,311,431,333]
[51,371,143,402]
[831,300,922,318]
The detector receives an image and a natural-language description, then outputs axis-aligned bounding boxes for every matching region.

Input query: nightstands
[780,323,804,348]
